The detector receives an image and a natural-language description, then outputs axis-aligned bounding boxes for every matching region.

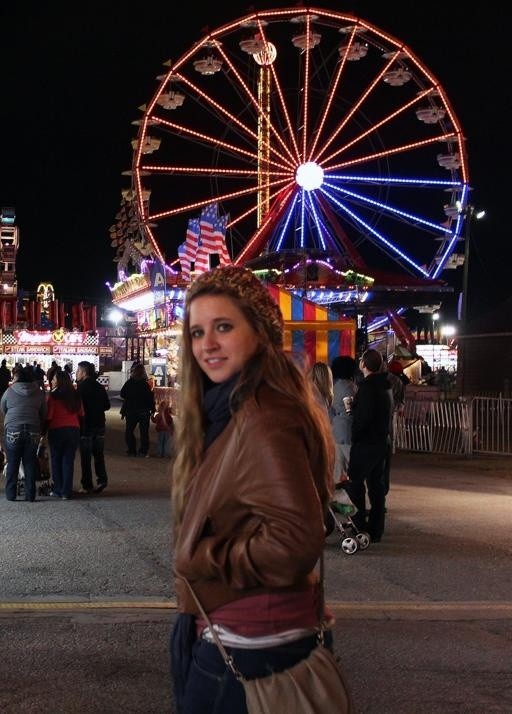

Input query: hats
[188,265,284,335]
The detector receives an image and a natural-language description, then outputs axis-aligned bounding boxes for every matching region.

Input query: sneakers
[77,485,96,494]
[96,482,109,494]
[48,492,71,500]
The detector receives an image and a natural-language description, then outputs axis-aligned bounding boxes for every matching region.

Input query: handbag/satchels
[239,647,349,713]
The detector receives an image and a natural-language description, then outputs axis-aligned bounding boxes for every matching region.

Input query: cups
[342,396,353,412]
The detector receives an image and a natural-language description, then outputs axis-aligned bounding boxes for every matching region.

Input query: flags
[177,203,232,282]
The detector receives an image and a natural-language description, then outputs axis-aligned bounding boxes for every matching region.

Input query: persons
[310,349,446,541]
[0,360,175,502]
[163,266,337,714]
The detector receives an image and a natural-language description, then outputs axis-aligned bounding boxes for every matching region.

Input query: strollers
[18,434,56,498]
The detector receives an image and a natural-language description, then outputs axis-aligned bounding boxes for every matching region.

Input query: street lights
[444,327,454,371]
[321,457,375,559]
[431,312,440,371]
[454,197,488,391]
